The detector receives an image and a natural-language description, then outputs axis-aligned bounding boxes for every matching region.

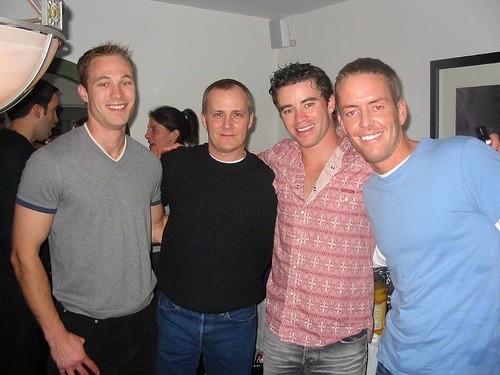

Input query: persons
[335,58,500,375]
[11,43,168,375]
[145,106,199,274]
[158,63,500,375]
[155,79,277,375]
[0,79,89,375]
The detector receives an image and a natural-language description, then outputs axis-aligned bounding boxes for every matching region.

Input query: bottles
[476,126,492,146]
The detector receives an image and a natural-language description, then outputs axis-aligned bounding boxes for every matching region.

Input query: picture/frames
[430,52,500,139]
